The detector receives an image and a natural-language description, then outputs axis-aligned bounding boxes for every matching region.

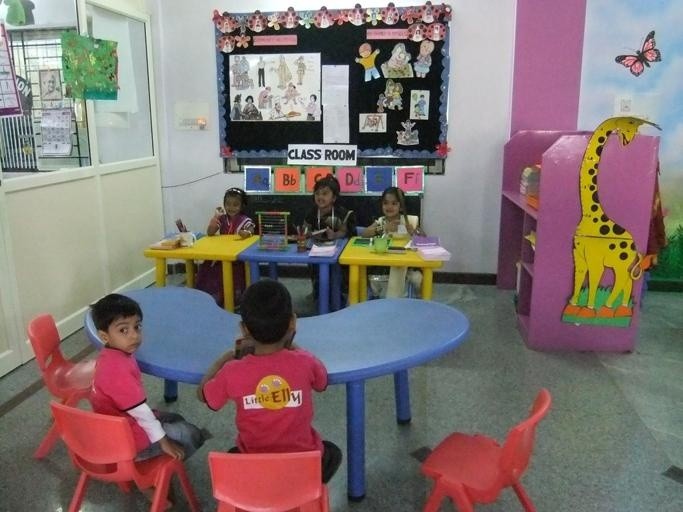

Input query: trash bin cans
[368,275,405,297]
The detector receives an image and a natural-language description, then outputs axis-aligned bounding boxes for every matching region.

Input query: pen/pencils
[375,230,384,238]
[176,219,187,232]
[293,224,309,236]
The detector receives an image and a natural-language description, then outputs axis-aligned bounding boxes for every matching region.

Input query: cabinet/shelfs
[496,132,658,356]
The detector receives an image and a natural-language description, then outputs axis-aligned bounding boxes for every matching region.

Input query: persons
[87,293,202,509]
[362,186,421,298]
[194,278,341,485]
[197,188,255,304]
[301,174,357,299]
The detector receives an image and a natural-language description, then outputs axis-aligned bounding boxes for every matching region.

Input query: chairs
[419,387,552,512]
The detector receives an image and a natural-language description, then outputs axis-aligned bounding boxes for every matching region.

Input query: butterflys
[614,30,662,77]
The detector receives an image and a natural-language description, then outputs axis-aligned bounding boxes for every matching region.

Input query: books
[412,234,451,262]
[150,232,198,250]
[517,157,549,212]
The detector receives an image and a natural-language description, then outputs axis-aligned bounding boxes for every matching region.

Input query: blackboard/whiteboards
[212,4,450,175]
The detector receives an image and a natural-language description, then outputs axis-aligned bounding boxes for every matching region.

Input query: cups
[297,236,307,252]
[372,235,392,254]
[179,231,196,249]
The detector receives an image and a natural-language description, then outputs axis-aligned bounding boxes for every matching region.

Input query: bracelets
[243,227,252,236]
[379,223,386,231]
